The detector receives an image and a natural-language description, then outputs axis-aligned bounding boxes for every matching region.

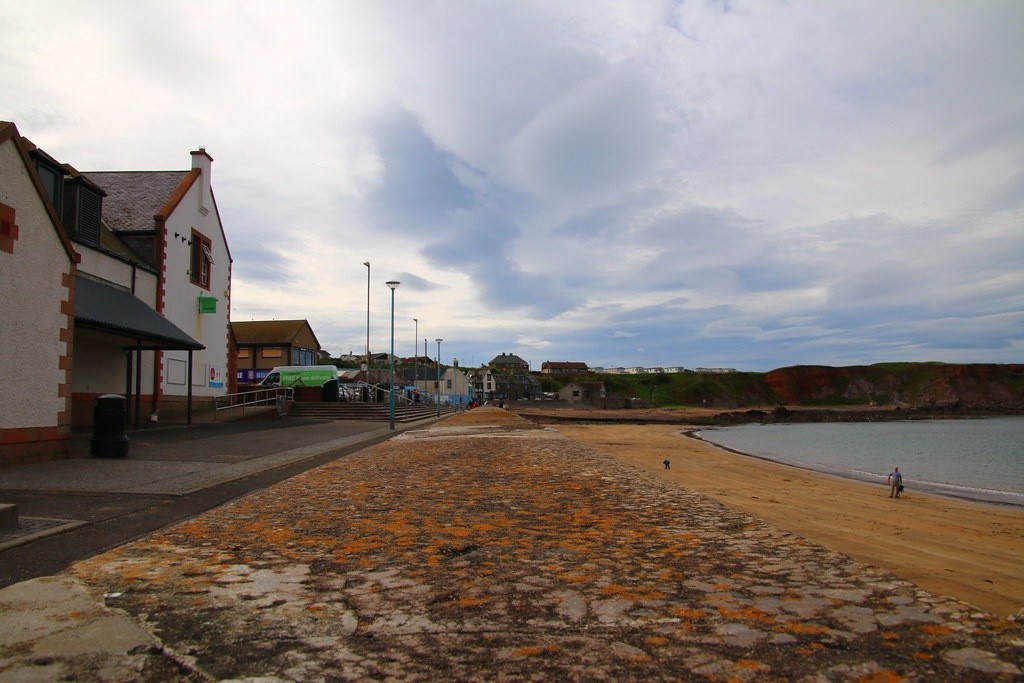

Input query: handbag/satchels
[898,484,904,491]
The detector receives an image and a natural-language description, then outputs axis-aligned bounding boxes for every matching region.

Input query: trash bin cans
[90,394,130,457]
[360,387,369,402]
[373,389,384,402]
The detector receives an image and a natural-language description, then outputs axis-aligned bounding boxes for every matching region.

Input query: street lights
[363,260,371,380]
[385,280,401,430]
[413,318,418,390]
[435,338,443,417]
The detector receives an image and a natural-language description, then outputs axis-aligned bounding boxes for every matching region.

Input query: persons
[663,460,670,469]
[888,467,902,498]
[407,389,479,410]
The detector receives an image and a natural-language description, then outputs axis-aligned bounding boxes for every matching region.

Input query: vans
[257,365,338,388]
